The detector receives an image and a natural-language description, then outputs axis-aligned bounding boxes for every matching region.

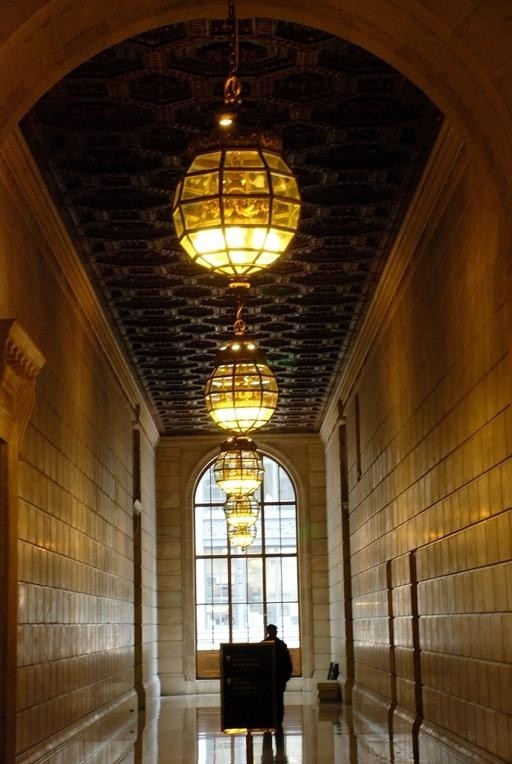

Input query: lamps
[212,434,267,501]
[170,2,300,295]
[222,495,261,531]
[225,524,258,551]
[201,296,281,437]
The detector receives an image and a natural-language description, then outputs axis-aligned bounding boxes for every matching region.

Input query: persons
[262,624,294,736]
